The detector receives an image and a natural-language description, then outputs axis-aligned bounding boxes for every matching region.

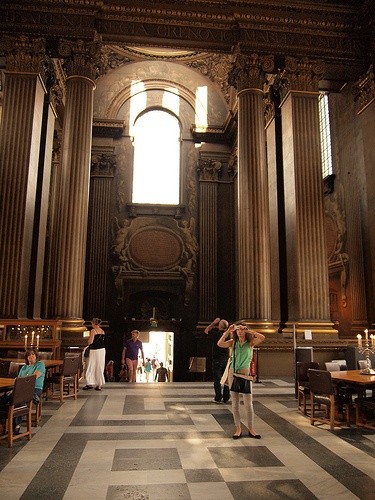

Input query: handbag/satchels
[219,357,233,389]
[84,347,90,358]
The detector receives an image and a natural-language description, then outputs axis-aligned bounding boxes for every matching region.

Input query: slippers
[95,387,102,391]
[82,385,94,390]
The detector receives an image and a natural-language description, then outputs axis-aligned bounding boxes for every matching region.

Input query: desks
[326,369,375,426]
[45,372,77,402]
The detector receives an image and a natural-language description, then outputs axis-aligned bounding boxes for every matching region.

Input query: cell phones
[235,326,240,329]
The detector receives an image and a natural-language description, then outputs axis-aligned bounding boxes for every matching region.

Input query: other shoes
[248,431,262,439]
[233,431,242,440]
[212,398,229,404]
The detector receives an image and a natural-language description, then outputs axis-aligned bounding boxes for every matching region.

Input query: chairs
[354,397,375,430]
[328,358,372,370]
[307,369,352,430]
[296,360,325,416]
[0,373,38,446]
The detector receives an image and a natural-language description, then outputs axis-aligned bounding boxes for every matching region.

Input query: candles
[357,328,375,355]
[23,330,40,348]
[0,359,65,372]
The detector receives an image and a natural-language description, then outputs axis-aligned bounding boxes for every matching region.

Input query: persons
[123,330,145,383]
[0,348,45,440]
[204,317,231,403]
[137,354,168,382]
[82,318,106,391]
[216,321,265,440]
